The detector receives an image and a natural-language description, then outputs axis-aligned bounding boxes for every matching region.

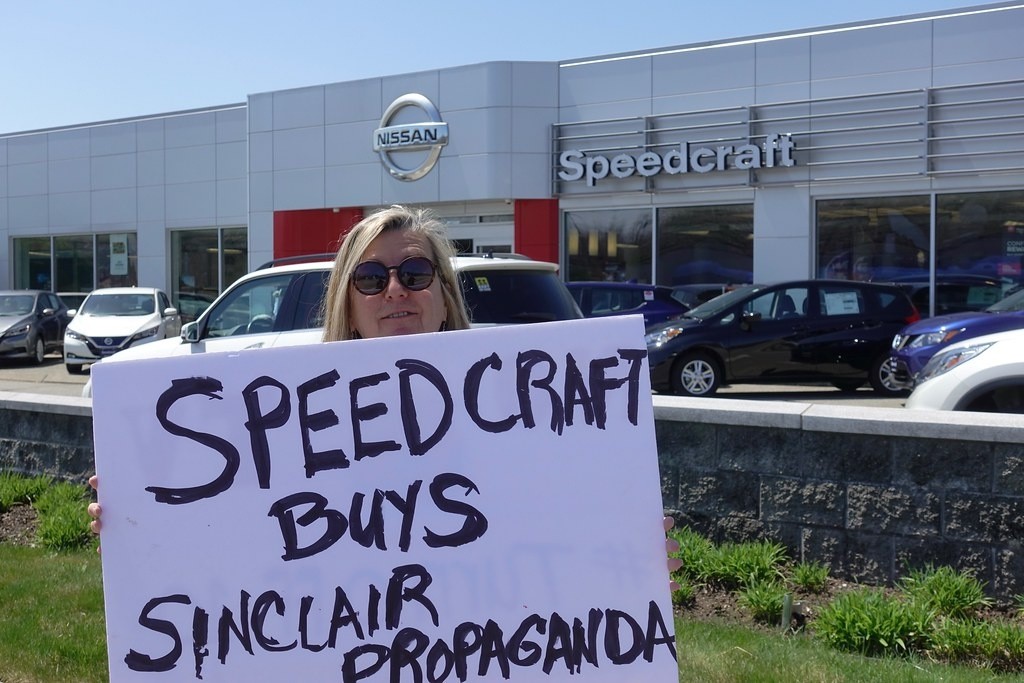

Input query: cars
[62,285,183,375]
[903,328,1024,414]
[890,287,1024,381]
[642,276,920,396]
[866,271,1024,319]
[0,288,74,368]
[564,277,688,332]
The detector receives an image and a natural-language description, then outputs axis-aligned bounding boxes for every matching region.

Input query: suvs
[96,246,583,360]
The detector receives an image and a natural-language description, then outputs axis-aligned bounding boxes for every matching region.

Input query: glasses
[350,256,438,296]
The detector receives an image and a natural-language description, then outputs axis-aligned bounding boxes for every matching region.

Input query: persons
[88,205,681,595]
